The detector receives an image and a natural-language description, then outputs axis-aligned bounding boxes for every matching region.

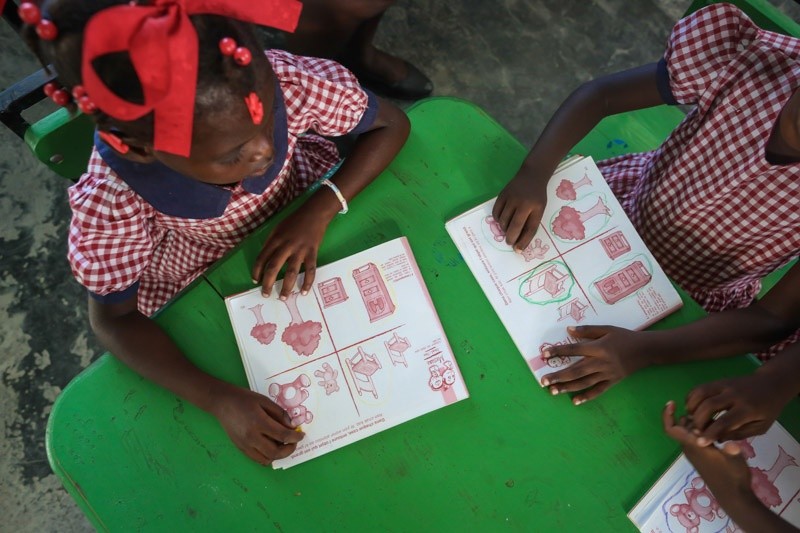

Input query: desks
[46,97,800,533]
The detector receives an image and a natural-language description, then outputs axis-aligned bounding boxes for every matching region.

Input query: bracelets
[321,178,349,214]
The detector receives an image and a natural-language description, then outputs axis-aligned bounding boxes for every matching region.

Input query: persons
[492,3,800,533]
[0,0,433,468]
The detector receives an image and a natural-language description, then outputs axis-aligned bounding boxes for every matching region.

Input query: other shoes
[354,49,433,99]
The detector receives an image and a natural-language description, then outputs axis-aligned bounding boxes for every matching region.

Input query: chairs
[565,1,800,300]
[0,65,94,183]
